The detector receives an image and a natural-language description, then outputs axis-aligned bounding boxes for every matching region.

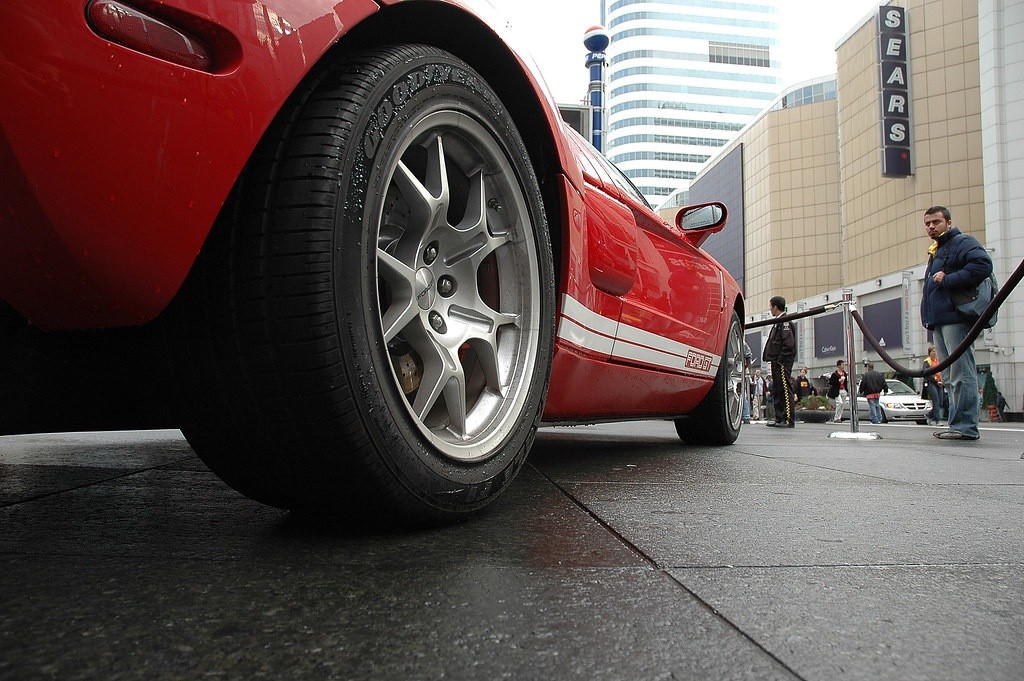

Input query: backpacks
[939,233,999,328]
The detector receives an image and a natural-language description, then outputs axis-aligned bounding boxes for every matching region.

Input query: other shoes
[745,419,749,423]
[766,418,782,426]
[933,429,979,440]
[775,419,795,428]
[835,420,842,422]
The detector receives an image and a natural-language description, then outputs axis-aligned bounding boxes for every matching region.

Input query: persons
[655,252,706,334]
[763,296,797,428]
[922,346,944,427]
[920,206,994,441]
[743,341,888,424]
[996,392,1010,422]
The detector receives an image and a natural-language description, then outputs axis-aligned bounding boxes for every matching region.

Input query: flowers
[796,395,834,411]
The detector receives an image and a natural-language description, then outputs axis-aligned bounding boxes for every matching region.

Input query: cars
[0,2,754,529]
[825,378,934,426]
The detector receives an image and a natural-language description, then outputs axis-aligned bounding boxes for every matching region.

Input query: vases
[796,411,836,423]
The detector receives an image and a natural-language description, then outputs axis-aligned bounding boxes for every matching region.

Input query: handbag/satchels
[828,388,835,399]
